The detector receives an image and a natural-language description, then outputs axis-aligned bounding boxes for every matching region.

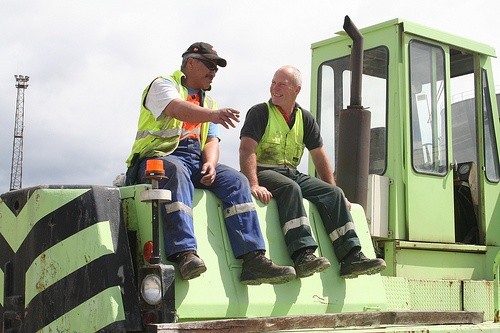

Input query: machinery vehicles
[0,14,499,333]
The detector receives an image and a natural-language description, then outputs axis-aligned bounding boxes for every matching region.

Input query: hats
[182,42,227,67]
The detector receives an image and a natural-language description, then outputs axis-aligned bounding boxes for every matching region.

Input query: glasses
[193,58,218,73]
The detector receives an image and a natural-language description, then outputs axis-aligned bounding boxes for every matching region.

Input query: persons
[240,65,387,279]
[125,42,295,285]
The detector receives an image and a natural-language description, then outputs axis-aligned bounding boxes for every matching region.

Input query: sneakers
[340,250,387,279]
[177,250,206,280]
[241,255,296,285]
[294,250,330,278]
